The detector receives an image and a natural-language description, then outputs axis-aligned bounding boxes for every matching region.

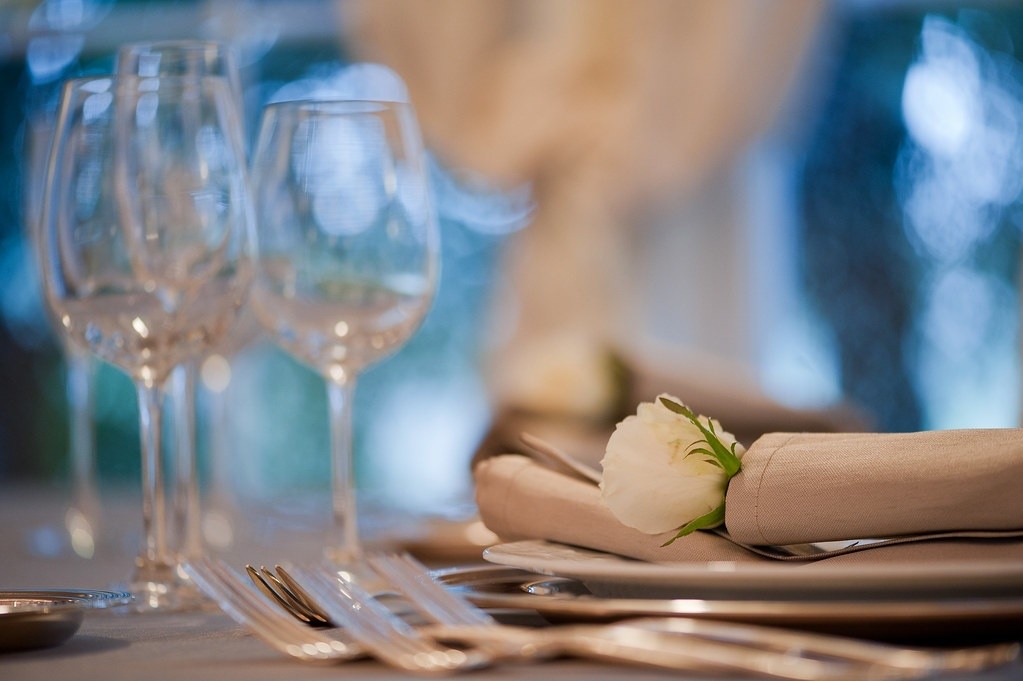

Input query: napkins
[474,429,1023,566]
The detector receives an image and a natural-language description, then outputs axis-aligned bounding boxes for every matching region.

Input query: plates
[482,539,1023,599]
[0,590,137,653]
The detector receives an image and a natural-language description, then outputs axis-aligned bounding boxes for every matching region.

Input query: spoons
[517,431,859,560]
[520,578,587,599]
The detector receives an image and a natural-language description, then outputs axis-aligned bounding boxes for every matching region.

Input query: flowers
[598,391,746,548]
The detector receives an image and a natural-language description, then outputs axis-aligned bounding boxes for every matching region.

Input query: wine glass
[40,78,258,629]
[249,101,440,584]
[118,44,245,586]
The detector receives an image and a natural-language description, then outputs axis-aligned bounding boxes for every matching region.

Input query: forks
[244,550,347,629]
[175,559,846,680]
[280,561,846,679]
[365,550,1015,668]
